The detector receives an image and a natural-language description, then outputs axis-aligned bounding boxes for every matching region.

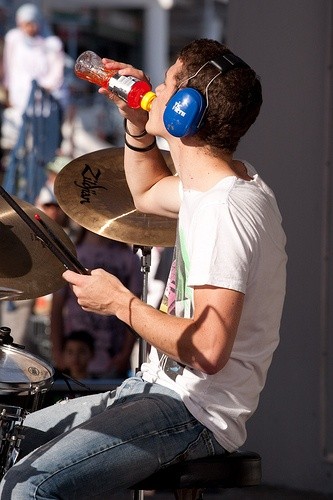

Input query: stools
[131,452,263,500]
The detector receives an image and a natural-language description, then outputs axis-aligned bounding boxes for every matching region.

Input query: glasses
[42,203,60,209]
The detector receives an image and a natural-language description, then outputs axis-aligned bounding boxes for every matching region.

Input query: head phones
[162,55,249,139]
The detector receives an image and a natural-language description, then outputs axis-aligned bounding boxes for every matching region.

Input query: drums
[0,338,54,482]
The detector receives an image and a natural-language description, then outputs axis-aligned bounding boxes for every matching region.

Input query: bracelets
[123,116,157,152]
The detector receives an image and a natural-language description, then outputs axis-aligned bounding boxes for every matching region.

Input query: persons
[0,37,286,500]
[0,3,144,393]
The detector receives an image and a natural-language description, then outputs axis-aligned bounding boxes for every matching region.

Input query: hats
[16,3,39,24]
[39,186,59,206]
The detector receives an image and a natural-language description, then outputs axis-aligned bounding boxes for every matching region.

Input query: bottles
[74,51,156,110]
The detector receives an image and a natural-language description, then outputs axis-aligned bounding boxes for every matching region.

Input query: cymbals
[53,145,177,250]
[0,192,77,303]
